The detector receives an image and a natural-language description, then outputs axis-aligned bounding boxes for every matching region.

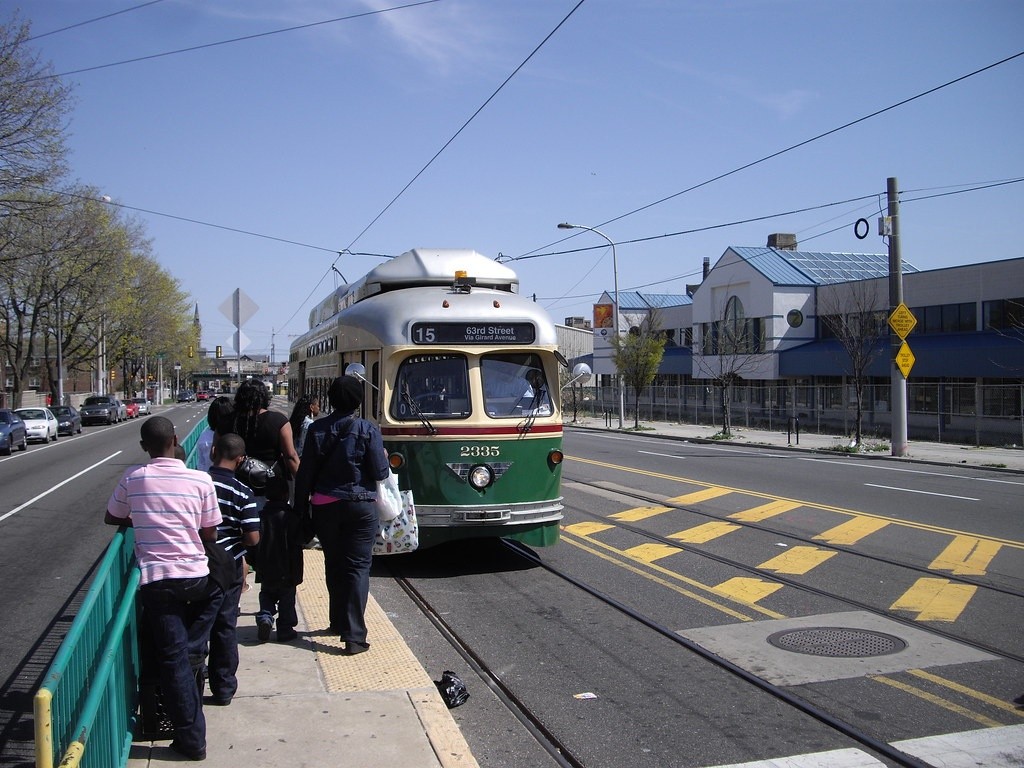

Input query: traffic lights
[110,370,116,380]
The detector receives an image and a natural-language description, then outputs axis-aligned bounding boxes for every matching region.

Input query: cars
[114,400,128,422]
[45,406,83,435]
[177,387,230,404]
[0,408,29,456]
[119,399,141,418]
[13,407,59,444]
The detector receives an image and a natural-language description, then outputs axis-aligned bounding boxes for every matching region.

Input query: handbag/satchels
[371,490,418,555]
[236,455,276,491]
[376,466,402,521]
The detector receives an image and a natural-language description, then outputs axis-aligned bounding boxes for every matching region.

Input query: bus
[286,248,593,553]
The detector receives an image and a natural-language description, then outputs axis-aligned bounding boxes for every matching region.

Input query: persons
[293,374,389,653]
[486,356,536,398]
[105,415,225,761]
[205,432,259,706]
[197,379,321,642]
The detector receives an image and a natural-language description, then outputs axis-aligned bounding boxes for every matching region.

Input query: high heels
[346,641,370,654]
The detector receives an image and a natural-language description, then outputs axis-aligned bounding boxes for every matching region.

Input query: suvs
[79,395,119,426]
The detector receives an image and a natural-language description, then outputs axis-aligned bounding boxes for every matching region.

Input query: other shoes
[258,619,271,641]
[277,629,297,642]
[217,698,231,705]
[169,742,206,760]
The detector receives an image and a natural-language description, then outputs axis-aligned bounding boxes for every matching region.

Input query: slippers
[244,583,252,593]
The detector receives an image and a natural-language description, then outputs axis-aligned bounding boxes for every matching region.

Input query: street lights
[557,222,624,429]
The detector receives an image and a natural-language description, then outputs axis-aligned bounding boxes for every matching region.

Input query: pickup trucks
[131,397,153,415]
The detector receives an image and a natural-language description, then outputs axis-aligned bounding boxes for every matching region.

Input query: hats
[328,376,363,411]
